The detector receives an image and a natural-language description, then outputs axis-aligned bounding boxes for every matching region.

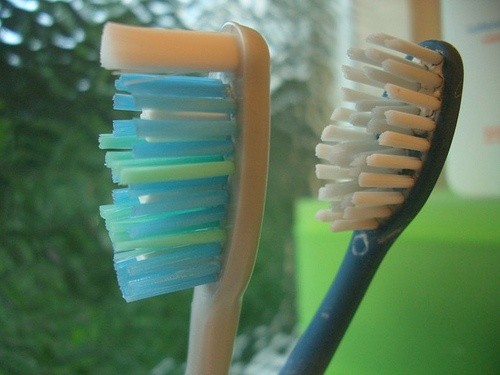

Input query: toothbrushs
[278,32,464,375]
[99,20,270,375]
